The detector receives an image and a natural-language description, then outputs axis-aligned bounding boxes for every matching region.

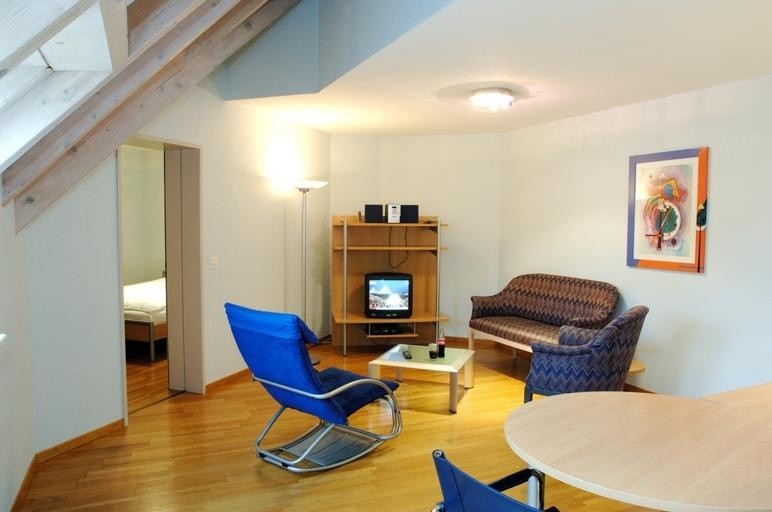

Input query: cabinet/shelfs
[331,213,450,357]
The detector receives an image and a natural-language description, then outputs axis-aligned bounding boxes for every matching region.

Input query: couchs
[467,273,619,361]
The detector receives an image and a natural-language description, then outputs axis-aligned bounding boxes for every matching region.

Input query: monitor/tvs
[364,272,412,319]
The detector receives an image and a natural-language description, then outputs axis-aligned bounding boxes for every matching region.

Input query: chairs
[426,447,556,512]
[524,306,649,403]
[223,302,403,474]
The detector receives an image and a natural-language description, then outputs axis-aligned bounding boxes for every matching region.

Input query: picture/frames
[626,146,710,274]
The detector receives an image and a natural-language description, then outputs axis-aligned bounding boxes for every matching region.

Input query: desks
[503,383,772,512]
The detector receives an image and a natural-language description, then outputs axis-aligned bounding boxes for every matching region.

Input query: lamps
[292,179,329,366]
[467,87,515,113]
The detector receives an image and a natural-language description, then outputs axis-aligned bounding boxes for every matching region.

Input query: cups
[429,343,438,360]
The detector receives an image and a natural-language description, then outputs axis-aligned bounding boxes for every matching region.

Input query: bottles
[438,328,446,358]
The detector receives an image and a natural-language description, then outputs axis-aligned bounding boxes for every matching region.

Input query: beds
[122,270,167,361]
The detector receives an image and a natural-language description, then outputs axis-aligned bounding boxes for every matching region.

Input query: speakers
[365,204,383,223]
[400,205,419,223]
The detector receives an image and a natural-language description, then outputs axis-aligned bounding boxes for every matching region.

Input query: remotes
[403,351,412,360]
